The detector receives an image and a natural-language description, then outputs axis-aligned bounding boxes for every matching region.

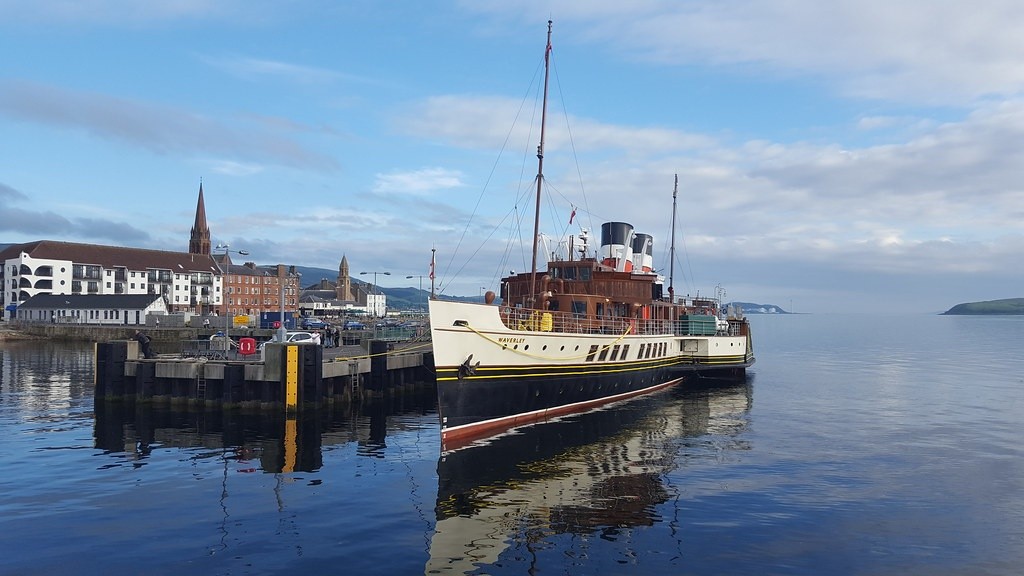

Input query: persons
[320,325,340,348]
[203,318,211,329]
[155,317,160,329]
[130,329,157,359]
[310,329,321,345]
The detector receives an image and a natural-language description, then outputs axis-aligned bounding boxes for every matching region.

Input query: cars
[344,320,367,331]
[398,320,424,331]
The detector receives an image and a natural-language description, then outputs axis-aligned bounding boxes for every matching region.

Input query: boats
[426,12,755,456]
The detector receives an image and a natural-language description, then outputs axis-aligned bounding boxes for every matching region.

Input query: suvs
[300,318,330,330]
[376,318,400,330]
[260,332,312,349]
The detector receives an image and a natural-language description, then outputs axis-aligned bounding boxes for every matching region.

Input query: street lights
[406,276,430,342]
[213,243,250,360]
[360,272,391,340]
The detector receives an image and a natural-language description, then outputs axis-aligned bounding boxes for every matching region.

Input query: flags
[429,256,436,278]
